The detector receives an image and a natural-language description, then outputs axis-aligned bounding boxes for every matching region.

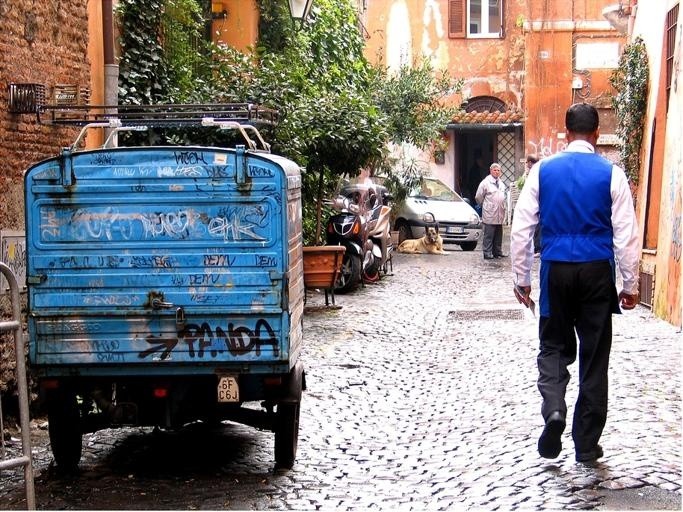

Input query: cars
[372,174,483,251]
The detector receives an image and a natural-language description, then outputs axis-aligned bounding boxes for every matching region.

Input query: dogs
[396,223,450,255]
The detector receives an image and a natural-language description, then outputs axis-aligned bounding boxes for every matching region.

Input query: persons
[523,152,541,258]
[507,101,642,466]
[473,161,509,260]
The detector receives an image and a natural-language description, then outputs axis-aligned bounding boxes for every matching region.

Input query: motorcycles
[322,182,393,291]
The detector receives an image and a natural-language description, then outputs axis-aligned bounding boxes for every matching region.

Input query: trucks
[20,100,314,486]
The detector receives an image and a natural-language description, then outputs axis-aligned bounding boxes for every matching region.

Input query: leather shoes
[576,448,603,462]
[538,411,566,459]
[484,254,508,259]
